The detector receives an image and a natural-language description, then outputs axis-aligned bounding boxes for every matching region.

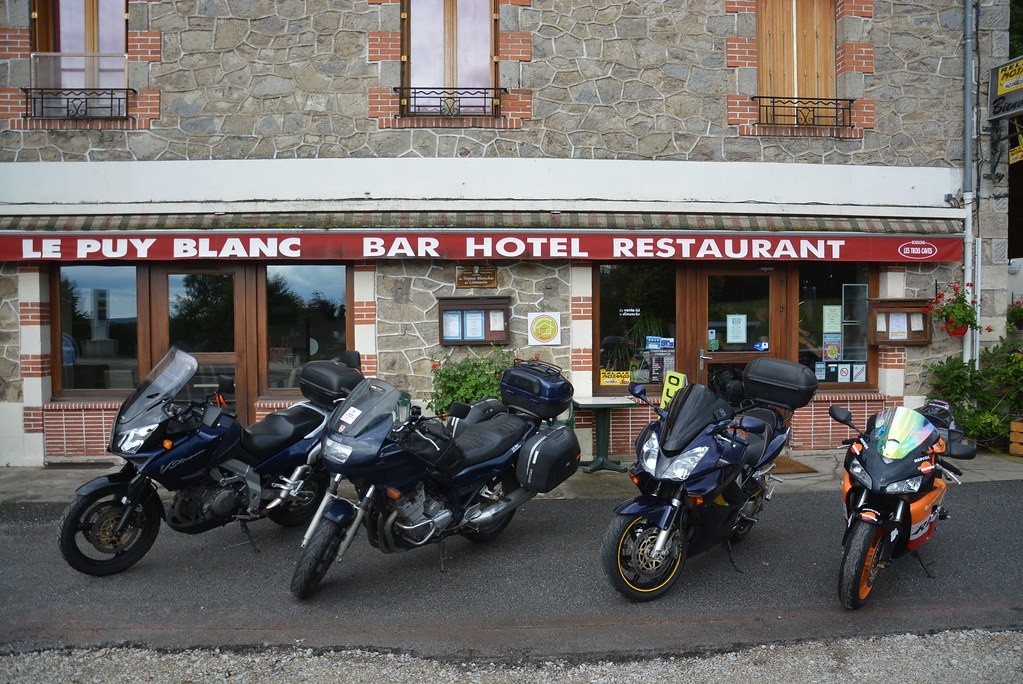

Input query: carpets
[760,457,819,475]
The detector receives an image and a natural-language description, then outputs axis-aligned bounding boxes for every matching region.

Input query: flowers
[923,277,995,335]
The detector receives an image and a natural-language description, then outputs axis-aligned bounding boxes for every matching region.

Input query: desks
[574,396,637,474]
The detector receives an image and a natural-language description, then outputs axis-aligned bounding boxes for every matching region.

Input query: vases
[945,324,968,337]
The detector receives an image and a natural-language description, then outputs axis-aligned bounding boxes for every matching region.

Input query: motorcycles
[597,356,818,598]
[291,350,583,600]
[56,344,365,576]
[825,397,977,612]
